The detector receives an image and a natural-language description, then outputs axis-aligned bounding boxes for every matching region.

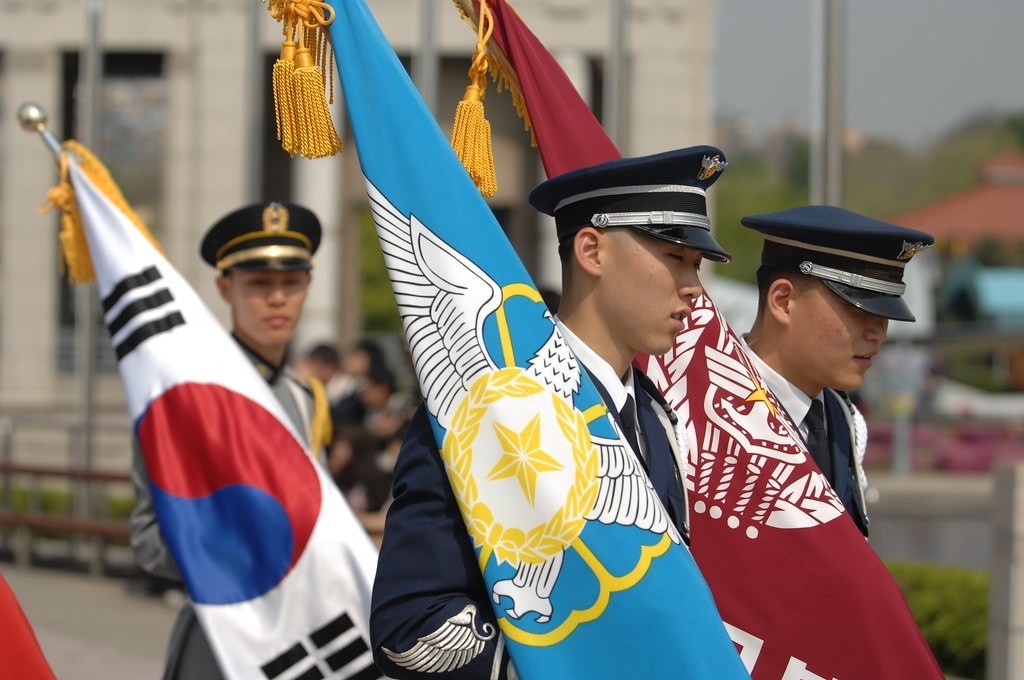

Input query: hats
[740,205,936,322]
[200,199,322,272]
[528,145,731,264]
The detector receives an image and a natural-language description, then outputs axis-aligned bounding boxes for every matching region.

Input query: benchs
[0,460,140,578]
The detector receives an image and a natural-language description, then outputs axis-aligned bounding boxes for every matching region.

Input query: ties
[804,399,831,489]
[618,394,650,479]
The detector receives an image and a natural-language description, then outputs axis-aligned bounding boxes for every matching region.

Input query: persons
[738,205,933,543]
[124,203,331,680]
[367,145,730,680]
[291,336,420,552]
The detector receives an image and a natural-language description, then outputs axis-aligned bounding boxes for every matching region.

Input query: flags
[38,140,380,680]
[264,1,755,679]
[448,0,944,680]
